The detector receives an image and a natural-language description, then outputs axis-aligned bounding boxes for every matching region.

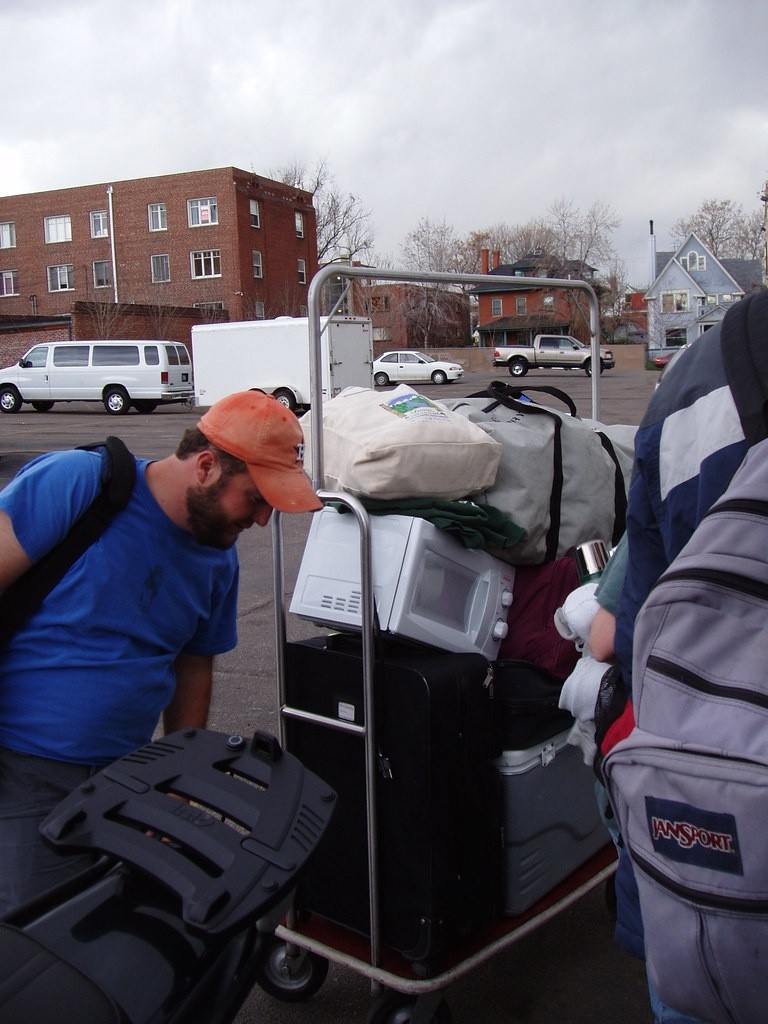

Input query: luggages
[287,629,508,978]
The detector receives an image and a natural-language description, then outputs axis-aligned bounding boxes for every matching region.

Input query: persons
[591,531,629,661]
[607,286,767,959]
[2,389,324,931]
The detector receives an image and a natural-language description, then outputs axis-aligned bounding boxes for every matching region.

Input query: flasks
[572,538,611,586]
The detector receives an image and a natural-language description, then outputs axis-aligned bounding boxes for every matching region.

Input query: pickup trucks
[492,334,615,377]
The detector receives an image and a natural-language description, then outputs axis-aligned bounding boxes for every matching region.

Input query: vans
[0,339,193,415]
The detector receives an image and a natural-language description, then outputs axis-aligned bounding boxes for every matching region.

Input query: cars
[374,350,464,386]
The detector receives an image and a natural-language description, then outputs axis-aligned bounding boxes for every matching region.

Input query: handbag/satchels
[433,378,641,566]
[299,384,504,503]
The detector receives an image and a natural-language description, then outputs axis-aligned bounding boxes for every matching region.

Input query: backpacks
[595,284,767,1023]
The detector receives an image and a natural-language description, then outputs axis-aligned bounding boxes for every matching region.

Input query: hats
[195,391,325,513]
